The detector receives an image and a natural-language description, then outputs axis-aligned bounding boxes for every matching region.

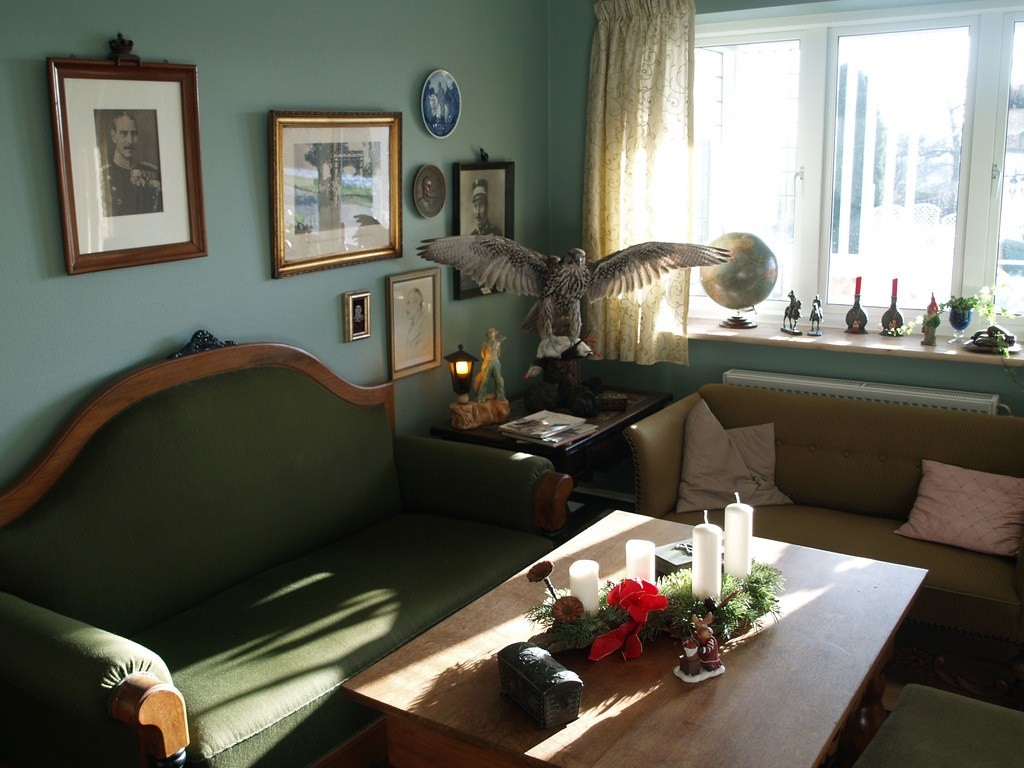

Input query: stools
[852,684,1024,768]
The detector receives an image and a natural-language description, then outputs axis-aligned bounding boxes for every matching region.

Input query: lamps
[443,344,480,405]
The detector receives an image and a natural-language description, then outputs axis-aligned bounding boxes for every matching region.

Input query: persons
[788,290,802,318]
[99,111,165,218]
[476,327,505,401]
[427,82,454,131]
[809,295,824,322]
[471,178,501,237]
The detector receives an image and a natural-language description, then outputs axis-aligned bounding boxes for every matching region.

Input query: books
[500,410,599,448]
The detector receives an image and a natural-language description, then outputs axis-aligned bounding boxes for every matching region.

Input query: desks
[339,510,932,768]
[430,383,674,507]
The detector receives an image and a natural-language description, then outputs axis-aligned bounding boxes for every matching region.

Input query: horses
[810,303,822,331]
[783,300,801,330]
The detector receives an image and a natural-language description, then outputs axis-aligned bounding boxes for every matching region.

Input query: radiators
[722,368,999,415]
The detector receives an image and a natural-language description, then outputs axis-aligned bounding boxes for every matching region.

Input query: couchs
[626,384,1024,700]
[0,330,573,768]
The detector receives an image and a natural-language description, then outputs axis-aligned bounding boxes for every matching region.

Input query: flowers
[886,283,1015,359]
[527,559,786,660]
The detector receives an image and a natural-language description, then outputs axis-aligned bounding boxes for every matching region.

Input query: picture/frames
[269,109,443,381]
[46,32,209,276]
[453,148,515,301]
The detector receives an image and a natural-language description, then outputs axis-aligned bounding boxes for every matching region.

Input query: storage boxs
[655,538,692,577]
[497,642,584,730]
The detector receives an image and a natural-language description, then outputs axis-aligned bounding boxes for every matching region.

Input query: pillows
[677,399,794,514]
[893,459,1024,557]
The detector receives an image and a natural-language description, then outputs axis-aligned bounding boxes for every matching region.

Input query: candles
[892,279,897,296]
[569,503,754,619]
[855,277,861,294]
